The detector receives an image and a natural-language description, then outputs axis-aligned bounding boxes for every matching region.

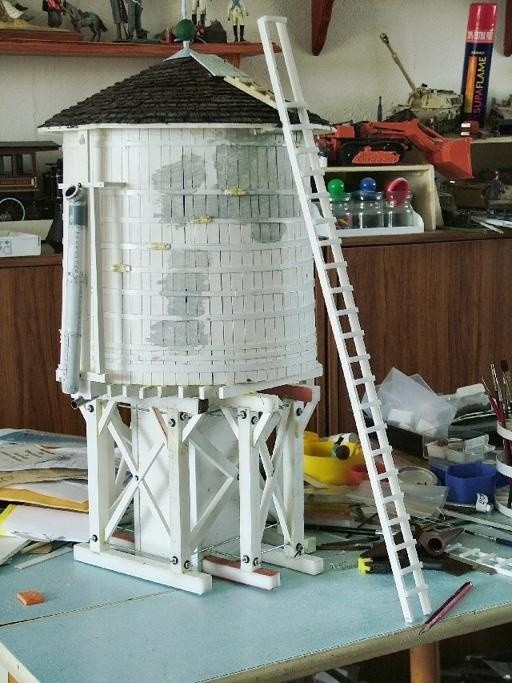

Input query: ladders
[256,11,433,626]
[446,541,512,578]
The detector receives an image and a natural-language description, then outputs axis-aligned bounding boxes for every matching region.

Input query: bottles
[462,2,499,129]
[329,177,414,230]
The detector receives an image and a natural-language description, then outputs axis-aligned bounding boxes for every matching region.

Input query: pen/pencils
[418,582,474,637]
[480,359,512,510]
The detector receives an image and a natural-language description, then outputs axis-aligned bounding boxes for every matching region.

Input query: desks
[1,508,511,681]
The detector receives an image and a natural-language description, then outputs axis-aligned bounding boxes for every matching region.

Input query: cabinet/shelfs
[338,239,511,436]
[3,263,90,437]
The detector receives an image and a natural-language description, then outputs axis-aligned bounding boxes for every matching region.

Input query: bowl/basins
[304,431,387,488]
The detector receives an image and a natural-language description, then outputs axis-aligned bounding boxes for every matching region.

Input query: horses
[63,3,108,43]
